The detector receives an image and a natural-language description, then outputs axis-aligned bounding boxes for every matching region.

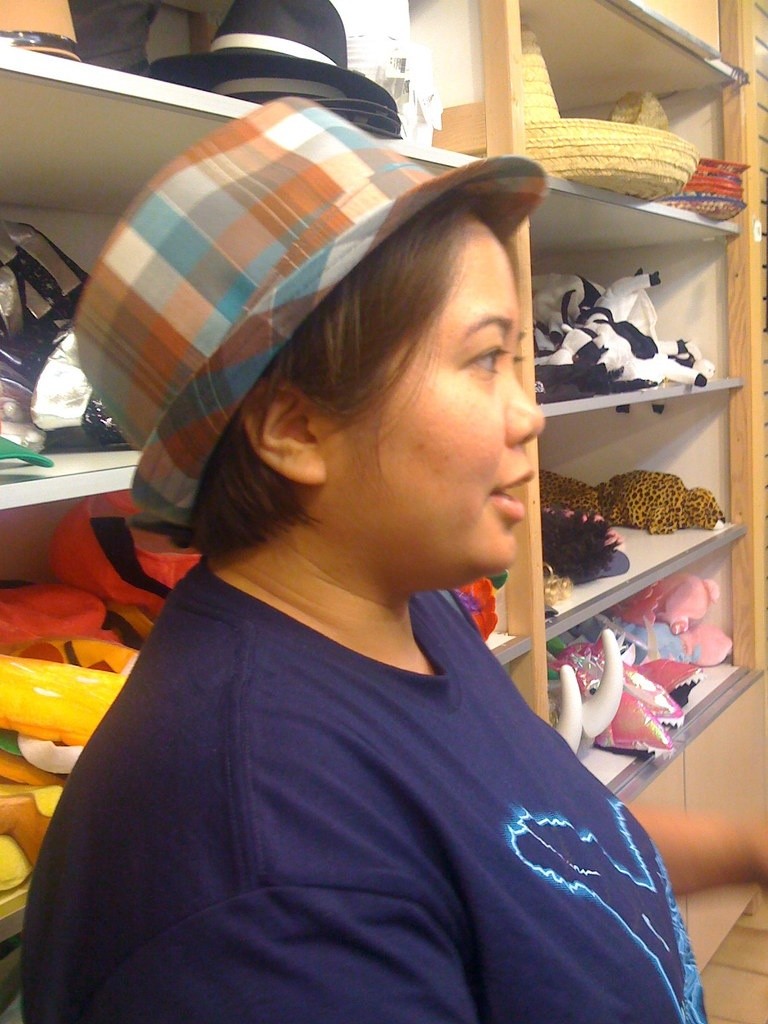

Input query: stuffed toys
[538,470,732,759]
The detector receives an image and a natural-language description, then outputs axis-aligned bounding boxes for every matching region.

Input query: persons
[18,91,708,1024]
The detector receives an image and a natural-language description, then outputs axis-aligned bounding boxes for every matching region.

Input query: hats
[74,96,547,528]
[0,436,54,468]
[0,0,752,223]
[537,507,736,757]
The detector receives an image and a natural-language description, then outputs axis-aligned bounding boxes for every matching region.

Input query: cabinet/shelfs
[0,0,536,1024]
[627,681,764,975]
[511,0,760,807]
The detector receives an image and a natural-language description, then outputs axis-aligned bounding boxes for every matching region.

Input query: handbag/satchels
[47,488,204,617]
[0,219,140,455]
